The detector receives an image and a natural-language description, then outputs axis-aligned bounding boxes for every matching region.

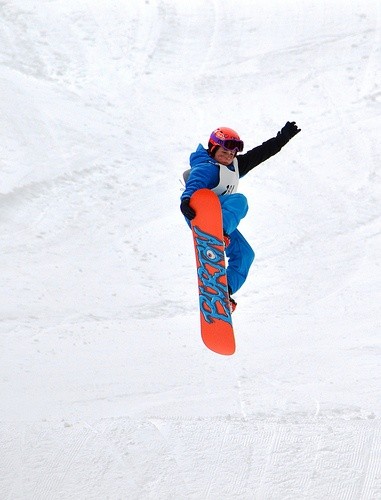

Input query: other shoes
[224,233,232,250]
[228,283,237,314]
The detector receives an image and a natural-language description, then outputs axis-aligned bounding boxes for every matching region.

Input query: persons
[179,120,301,315]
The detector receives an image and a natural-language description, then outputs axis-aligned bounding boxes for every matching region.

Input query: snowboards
[189,189,235,355]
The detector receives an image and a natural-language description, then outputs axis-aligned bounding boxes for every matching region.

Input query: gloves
[262,120,301,156]
[181,197,197,221]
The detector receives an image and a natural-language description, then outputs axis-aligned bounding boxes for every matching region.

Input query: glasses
[211,133,244,153]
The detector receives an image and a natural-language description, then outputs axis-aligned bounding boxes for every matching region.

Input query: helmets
[208,127,244,160]
